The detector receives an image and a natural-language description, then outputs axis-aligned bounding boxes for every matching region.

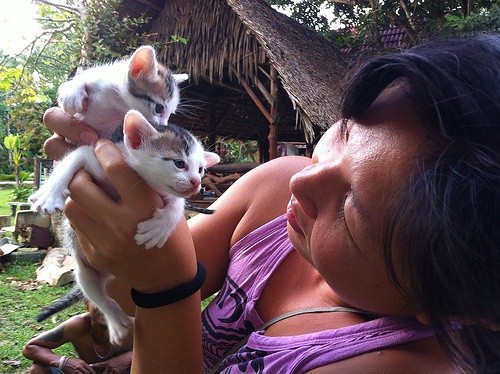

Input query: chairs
[1,210,39,245]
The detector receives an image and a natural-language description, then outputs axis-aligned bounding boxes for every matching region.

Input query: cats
[26,44,221,346]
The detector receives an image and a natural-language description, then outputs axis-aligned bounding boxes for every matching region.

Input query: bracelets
[131,263,207,308]
[59,356,69,370]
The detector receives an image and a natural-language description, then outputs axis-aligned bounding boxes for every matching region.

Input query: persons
[44,33,500,373]
[22,295,133,374]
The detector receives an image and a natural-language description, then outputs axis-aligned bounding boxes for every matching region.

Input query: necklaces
[89,329,113,358]
